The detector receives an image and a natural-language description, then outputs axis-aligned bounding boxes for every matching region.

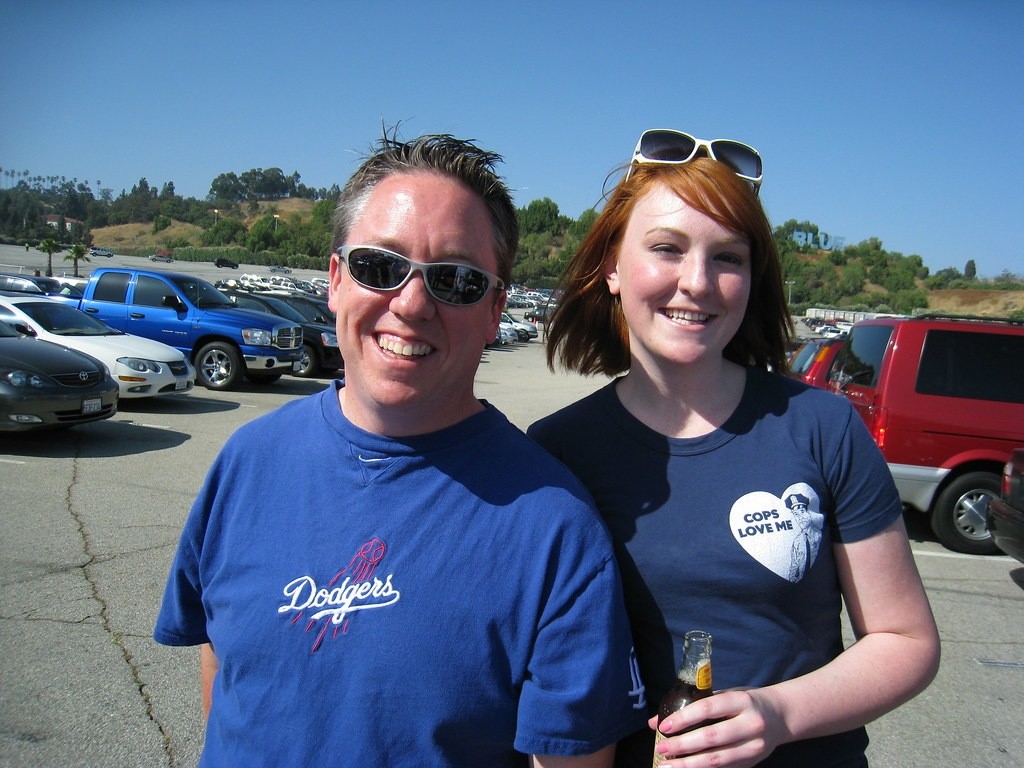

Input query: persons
[153,118,648,768]
[526,127,941,768]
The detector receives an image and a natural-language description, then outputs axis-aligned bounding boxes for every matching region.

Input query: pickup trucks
[7,266,307,391]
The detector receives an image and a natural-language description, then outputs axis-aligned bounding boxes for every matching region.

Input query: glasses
[337,244,505,306]
[625,128,763,195]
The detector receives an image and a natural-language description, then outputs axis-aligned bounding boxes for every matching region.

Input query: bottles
[651,630,714,768]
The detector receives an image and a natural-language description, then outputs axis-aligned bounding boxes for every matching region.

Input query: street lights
[213,209,219,227]
[273,214,281,244]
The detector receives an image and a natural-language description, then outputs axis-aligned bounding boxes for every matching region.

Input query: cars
[486,284,569,350]
[0,318,122,434]
[90,247,115,259]
[0,274,346,378]
[0,291,199,406]
[214,258,240,270]
[267,265,293,274]
[983,445,1024,567]
[149,255,173,264]
[782,317,854,389]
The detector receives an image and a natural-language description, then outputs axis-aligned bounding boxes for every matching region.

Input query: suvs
[824,314,1023,554]
